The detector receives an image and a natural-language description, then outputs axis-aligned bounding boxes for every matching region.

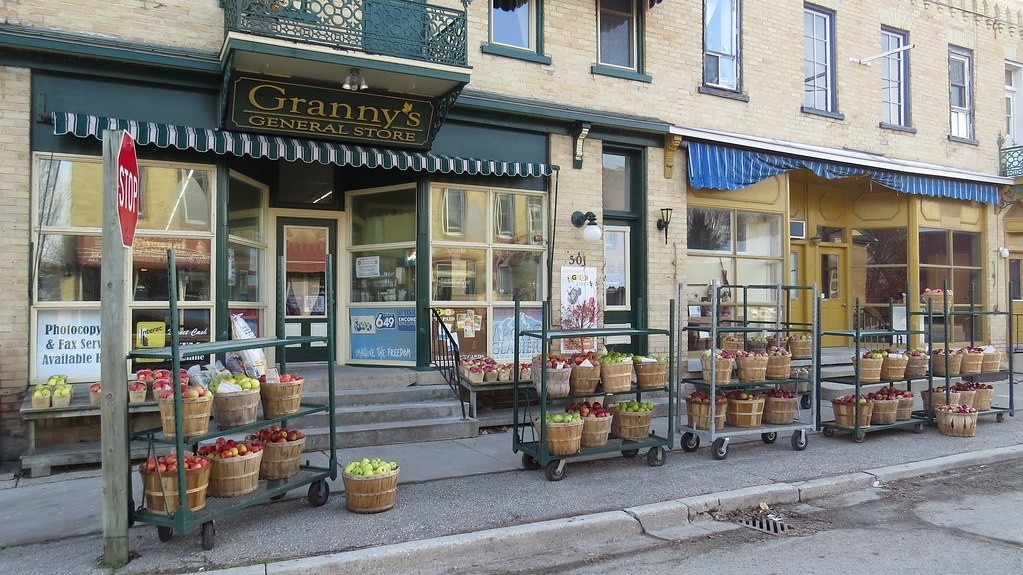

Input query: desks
[448,368,566,427]
[19,383,220,478]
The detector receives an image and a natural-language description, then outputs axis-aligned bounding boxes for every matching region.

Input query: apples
[925,288,952,294]
[144,426,305,472]
[33,375,73,397]
[131,368,300,398]
[790,367,807,372]
[90,383,101,392]
[860,347,985,359]
[460,357,533,374]
[837,381,993,412]
[535,351,666,369]
[689,388,796,401]
[343,458,397,476]
[537,399,653,422]
[701,335,811,357]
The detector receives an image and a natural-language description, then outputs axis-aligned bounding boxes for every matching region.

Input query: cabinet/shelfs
[513,279,1015,479]
[128,247,338,552]
[687,290,787,359]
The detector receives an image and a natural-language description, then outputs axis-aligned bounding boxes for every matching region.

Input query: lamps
[342,67,370,91]
[571,210,603,242]
[657,208,673,244]
[809,233,822,247]
[992,247,1009,258]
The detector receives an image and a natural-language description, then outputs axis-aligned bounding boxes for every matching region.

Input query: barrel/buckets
[530,292,1001,457]
[341,470,400,513]
[140,378,303,514]
